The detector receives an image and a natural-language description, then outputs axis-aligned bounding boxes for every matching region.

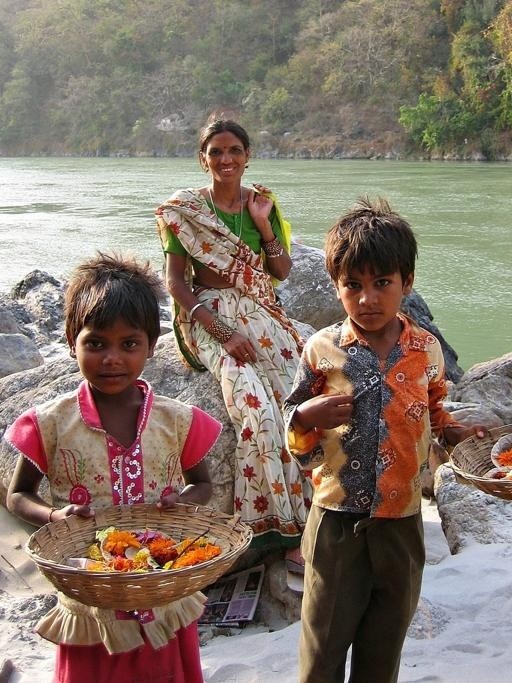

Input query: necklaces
[208,185,244,243]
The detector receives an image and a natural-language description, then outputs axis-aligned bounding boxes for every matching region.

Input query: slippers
[284,546,307,593]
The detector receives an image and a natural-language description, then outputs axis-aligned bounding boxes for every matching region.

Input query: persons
[3,249,226,683]
[281,191,490,682]
[154,107,325,599]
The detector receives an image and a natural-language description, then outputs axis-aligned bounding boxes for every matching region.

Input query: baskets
[25,502,254,609]
[449,423,512,501]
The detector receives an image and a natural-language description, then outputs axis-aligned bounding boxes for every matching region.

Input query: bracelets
[267,248,284,258]
[260,234,281,256]
[190,302,204,321]
[48,506,58,523]
[204,318,235,346]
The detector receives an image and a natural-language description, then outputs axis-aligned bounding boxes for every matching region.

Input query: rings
[243,353,250,358]
